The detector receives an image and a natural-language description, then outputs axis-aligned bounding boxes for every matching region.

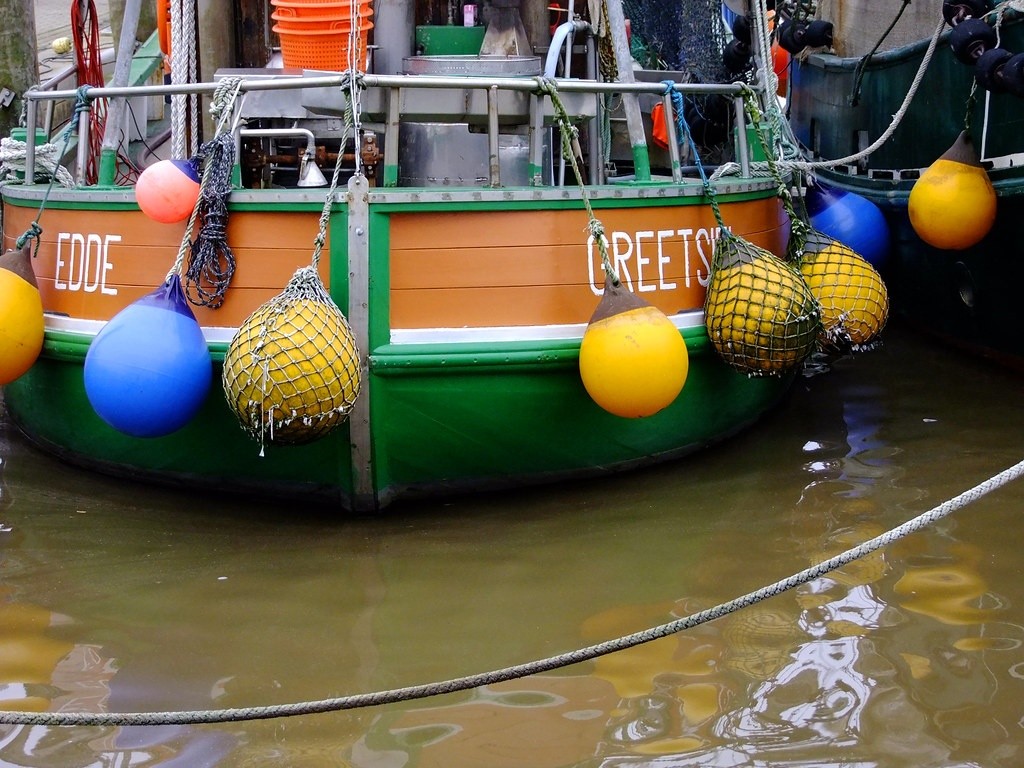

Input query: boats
[0,1,811,520]
[775,1,1024,354]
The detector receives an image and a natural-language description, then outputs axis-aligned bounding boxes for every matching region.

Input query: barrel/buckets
[402,55,552,187]
